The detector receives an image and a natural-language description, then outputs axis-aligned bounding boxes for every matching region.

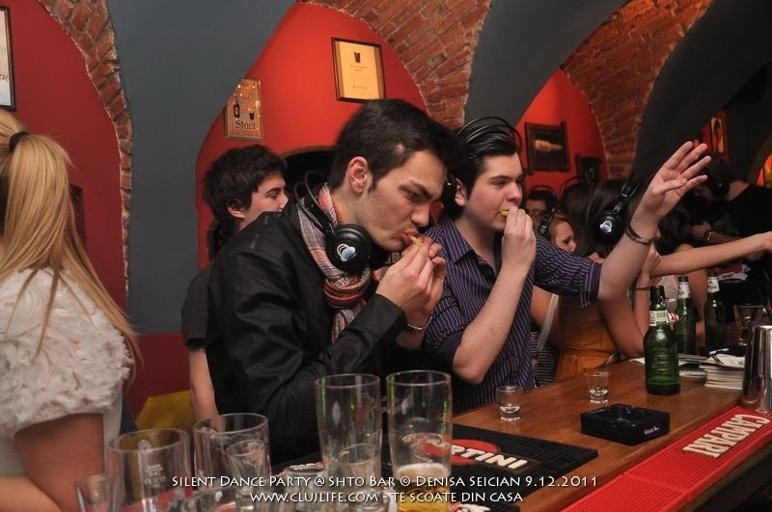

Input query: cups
[193,412,272,512]
[288,466,328,511]
[315,372,390,511]
[224,438,266,512]
[730,303,763,347]
[585,371,611,404]
[104,427,193,511]
[385,369,455,512]
[74,472,118,511]
[495,386,523,420]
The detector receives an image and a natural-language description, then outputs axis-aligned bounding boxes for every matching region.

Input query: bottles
[672,275,698,355]
[703,271,727,350]
[640,285,683,395]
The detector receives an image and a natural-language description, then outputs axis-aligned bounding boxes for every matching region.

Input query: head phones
[538,206,566,243]
[291,168,372,275]
[598,169,642,241]
[701,170,730,197]
[443,116,521,207]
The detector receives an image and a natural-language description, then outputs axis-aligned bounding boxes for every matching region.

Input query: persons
[181,98,772,484]
[0,106,144,512]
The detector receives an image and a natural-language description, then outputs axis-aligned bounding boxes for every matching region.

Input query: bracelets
[634,286,650,290]
[407,314,433,334]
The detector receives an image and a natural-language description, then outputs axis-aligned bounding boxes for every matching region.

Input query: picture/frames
[709,115,726,155]
[224,76,264,142]
[0,6,16,112]
[525,122,571,175]
[331,36,386,104]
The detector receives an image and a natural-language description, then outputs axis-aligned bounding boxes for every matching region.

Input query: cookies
[409,236,420,249]
[501,211,508,217]
